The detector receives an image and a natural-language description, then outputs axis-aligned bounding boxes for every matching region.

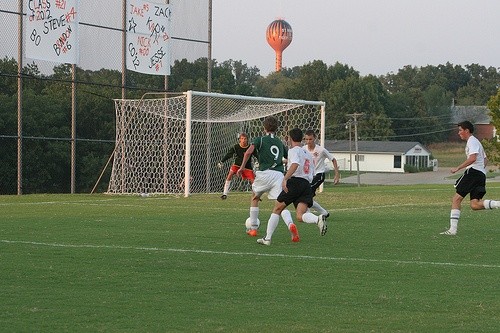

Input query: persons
[439,120,500,235]
[217,132,263,201]
[282,130,340,219]
[256,128,328,246]
[235,115,300,242]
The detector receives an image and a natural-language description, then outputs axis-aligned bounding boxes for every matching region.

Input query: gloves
[217,161,223,168]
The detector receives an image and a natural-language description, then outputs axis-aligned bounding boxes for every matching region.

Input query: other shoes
[439,230,456,235]
[221,195,227,199]
[325,212,330,218]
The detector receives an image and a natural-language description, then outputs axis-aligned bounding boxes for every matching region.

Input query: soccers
[246,217,260,230]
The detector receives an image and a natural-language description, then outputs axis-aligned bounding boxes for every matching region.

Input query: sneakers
[257,236,272,246]
[317,214,328,236]
[288,222,300,242]
[247,229,256,237]
